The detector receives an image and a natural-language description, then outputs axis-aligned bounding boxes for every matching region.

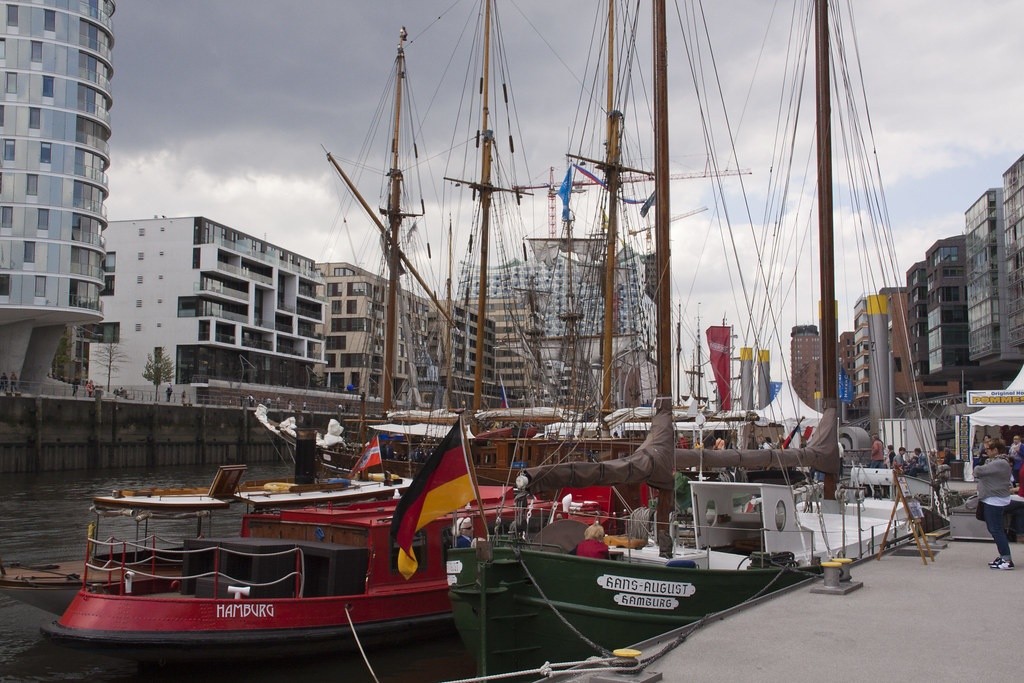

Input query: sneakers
[988,557,1005,565]
[990,560,1015,569]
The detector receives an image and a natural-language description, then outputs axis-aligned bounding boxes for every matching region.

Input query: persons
[72,375,81,396]
[452,517,474,548]
[676,432,729,451]
[61,375,65,382]
[87,380,95,397]
[0,372,8,392]
[248,393,307,410]
[10,371,17,392]
[759,434,786,450]
[838,441,844,478]
[869,433,929,476]
[576,523,609,560]
[942,447,955,466]
[182,390,186,403]
[972,433,1024,570]
[166,384,173,402]
[338,402,352,413]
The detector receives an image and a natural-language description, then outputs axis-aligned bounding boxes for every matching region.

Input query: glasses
[459,526,472,530]
[984,448,994,450]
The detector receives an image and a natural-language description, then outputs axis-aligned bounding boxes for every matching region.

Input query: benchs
[949,505,1012,541]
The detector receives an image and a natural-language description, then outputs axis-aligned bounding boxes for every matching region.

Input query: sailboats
[0,0,969,683]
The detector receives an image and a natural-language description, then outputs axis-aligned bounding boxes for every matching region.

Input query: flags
[390,418,477,582]
[352,433,381,479]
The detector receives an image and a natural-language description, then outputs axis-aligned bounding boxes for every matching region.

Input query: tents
[963,364,1024,482]
[718,376,824,474]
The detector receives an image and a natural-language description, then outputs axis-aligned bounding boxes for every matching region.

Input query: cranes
[512,165,753,239]
[630,205,709,255]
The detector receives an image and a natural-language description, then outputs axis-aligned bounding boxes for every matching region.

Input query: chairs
[665,560,700,569]
